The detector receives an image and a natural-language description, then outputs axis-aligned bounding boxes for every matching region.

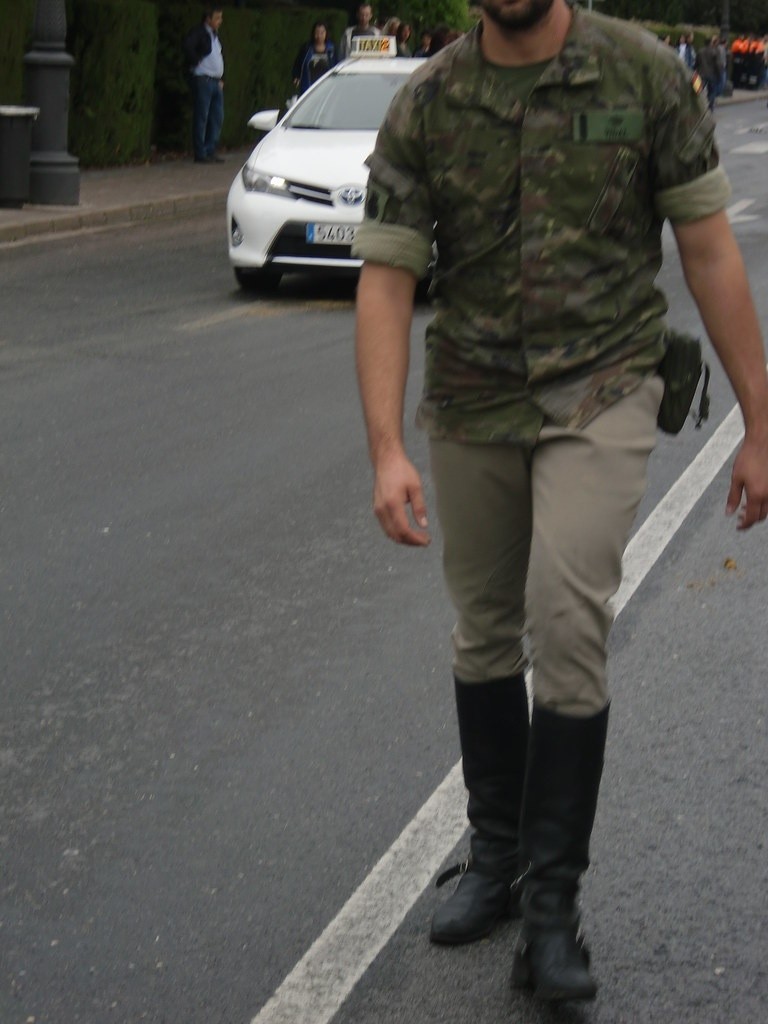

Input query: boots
[427,676,614,1003]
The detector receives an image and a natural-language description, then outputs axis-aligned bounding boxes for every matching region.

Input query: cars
[224,54,437,299]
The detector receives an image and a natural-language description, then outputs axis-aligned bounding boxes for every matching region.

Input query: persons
[339,0,767,998]
[170,7,228,165]
[291,23,337,102]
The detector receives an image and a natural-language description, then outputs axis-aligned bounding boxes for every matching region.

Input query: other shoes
[196,146,228,166]
